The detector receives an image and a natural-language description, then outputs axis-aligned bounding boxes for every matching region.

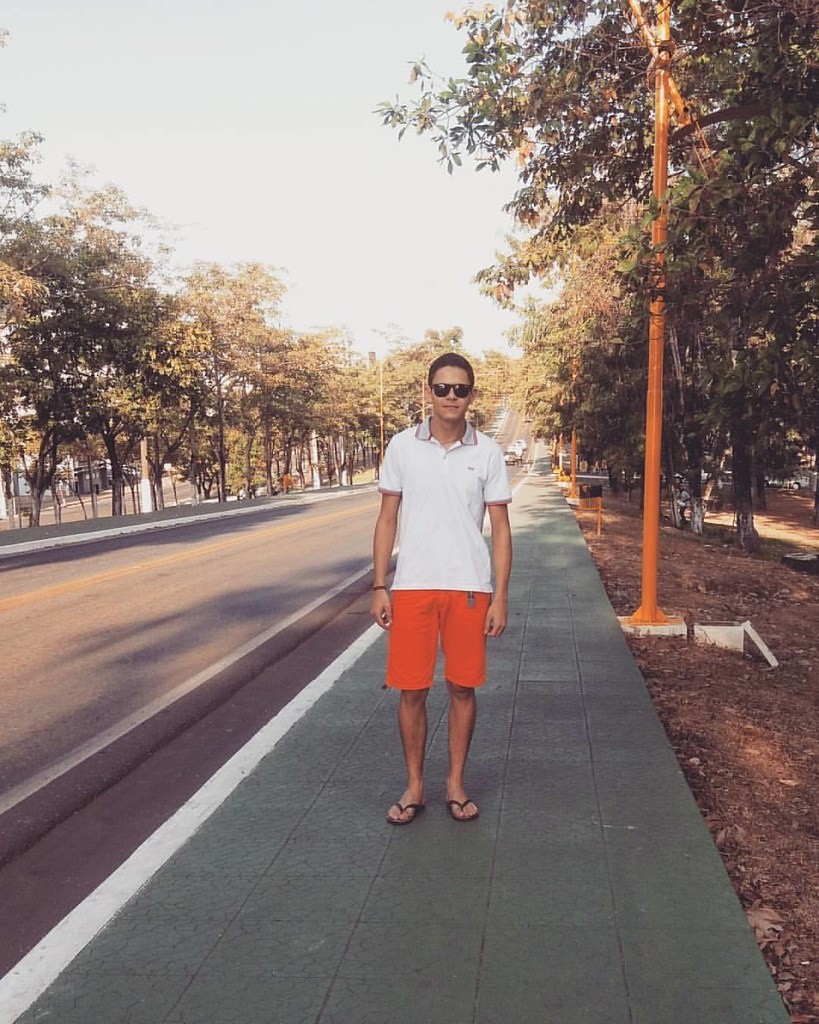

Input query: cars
[237,482,276,501]
[504,439,527,466]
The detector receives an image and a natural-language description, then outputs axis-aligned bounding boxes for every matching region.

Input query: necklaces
[442,441,457,444]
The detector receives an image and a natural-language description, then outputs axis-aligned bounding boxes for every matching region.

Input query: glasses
[430,383,471,398]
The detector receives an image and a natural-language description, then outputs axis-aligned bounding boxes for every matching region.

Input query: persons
[371,353,514,824]
[676,486,690,524]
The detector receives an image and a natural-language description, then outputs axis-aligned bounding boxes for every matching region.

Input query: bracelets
[372,585,388,591]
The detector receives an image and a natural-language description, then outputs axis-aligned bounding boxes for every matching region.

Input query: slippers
[385,802,425,825]
[446,798,479,821]
